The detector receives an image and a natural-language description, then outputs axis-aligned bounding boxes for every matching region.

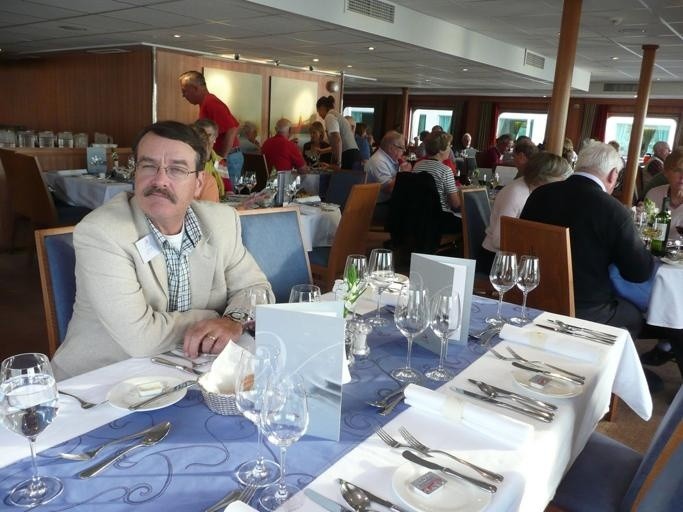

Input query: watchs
[226,310,251,328]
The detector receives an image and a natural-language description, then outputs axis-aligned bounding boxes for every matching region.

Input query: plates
[104,375,187,412]
[511,367,586,398]
[390,451,492,512]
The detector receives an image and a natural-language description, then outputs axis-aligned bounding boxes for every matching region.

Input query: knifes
[535,323,613,346]
[511,361,581,385]
[401,450,497,493]
[449,384,550,423]
[467,377,558,409]
[337,477,408,511]
[127,379,195,411]
[301,487,349,508]
[150,356,203,376]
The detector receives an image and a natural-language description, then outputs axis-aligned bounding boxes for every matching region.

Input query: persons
[354,121,371,164]
[176,70,242,196]
[46,121,275,382]
[478,135,515,173]
[413,131,431,158]
[642,141,671,190]
[456,134,477,158]
[643,148,683,241]
[262,117,309,175]
[474,151,578,273]
[302,121,331,168]
[184,123,218,203]
[344,115,370,168]
[512,139,539,181]
[415,125,455,164]
[193,119,234,196]
[502,136,531,167]
[235,121,263,156]
[317,97,361,170]
[411,133,468,253]
[518,140,652,342]
[365,130,412,267]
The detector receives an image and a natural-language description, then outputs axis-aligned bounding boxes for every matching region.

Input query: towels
[334,279,406,309]
[498,322,608,368]
[295,195,321,205]
[402,383,535,446]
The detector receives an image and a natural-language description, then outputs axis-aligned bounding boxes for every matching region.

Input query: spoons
[364,381,411,416]
[467,324,503,339]
[339,482,371,512]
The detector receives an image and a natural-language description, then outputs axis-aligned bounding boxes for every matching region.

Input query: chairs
[35,227,77,361]
[241,153,270,195]
[308,183,381,293]
[238,207,315,305]
[495,166,519,187]
[463,158,476,176]
[323,170,366,206]
[1,150,58,256]
[550,388,683,512]
[396,171,459,256]
[500,216,575,318]
[462,190,499,306]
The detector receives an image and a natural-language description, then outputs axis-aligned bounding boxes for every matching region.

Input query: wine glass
[641,212,658,246]
[485,252,518,324]
[287,283,320,304]
[243,170,256,193]
[510,253,540,327]
[0,351,64,509]
[257,370,309,512]
[341,254,370,322]
[233,335,285,487]
[237,286,269,332]
[489,172,499,199]
[233,175,245,197]
[388,287,431,386]
[424,293,460,382]
[367,247,394,327]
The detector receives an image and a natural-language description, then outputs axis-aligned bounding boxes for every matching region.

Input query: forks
[397,423,504,483]
[548,318,616,342]
[505,345,584,379]
[236,480,257,504]
[374,423,432,458]
[58,389,95,410]
[478,381,554,416]
[488,346,538,368]
[202,490,240,512]
[161,350,202,368]
[77,419,171,478]
[58,419,166,462]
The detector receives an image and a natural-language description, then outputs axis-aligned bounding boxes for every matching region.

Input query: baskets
[199,385,255,418]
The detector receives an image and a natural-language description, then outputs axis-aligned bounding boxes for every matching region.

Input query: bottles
[274,171,285,207]
[651,197,671,257]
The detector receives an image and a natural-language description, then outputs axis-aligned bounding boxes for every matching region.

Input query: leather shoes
[639,345,681,367]
[644,370,664,392]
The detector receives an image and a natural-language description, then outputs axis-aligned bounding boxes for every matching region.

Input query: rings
[206,334,216,340]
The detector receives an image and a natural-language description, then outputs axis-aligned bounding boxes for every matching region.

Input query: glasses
[392,143,406,150]
[133,159,202,183]
